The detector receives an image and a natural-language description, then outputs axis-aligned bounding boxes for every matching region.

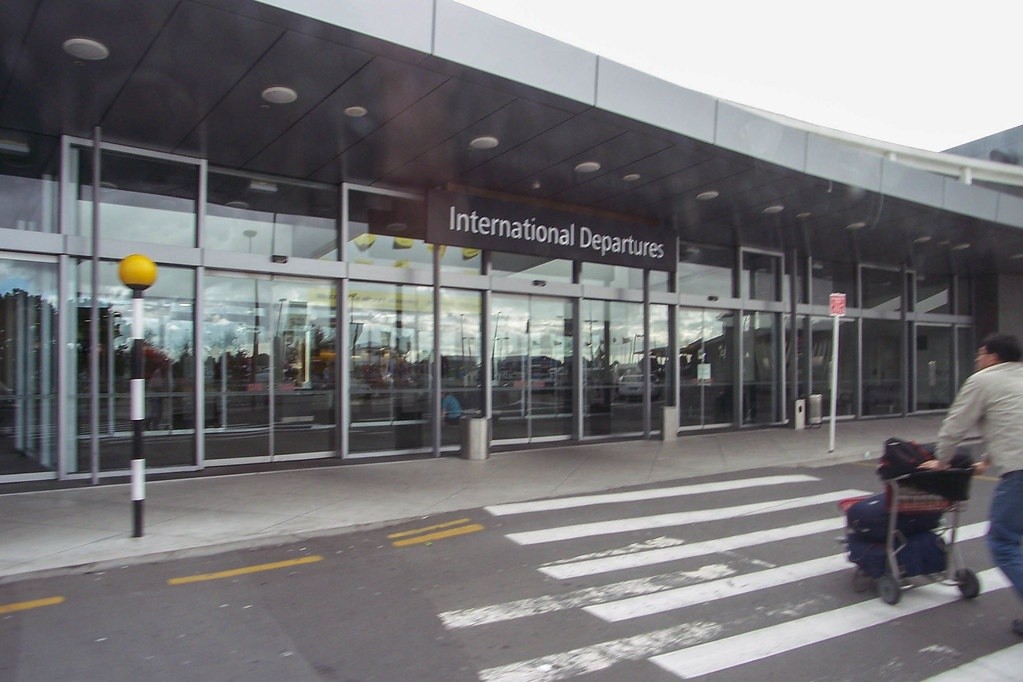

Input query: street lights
[349,293,357,323]
[496,336,509,367]
[461,337,468,371]
[467,336,476,372]
[276,297,287,337]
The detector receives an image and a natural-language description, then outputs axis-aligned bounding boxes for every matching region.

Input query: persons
[394,367,423,449]
[442,393,462,426]
[916,334,1023,637]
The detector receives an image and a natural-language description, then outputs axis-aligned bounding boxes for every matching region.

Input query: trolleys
[835,463,980,606]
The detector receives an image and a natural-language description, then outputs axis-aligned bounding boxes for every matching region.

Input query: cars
[617,373,662,399]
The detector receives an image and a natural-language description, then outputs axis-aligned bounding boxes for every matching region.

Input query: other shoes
[1010,619,1023,635]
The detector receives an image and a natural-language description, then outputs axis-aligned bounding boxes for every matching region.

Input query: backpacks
[876,437,973,501]
[846,531,946,578]
[845,492,942,540]
